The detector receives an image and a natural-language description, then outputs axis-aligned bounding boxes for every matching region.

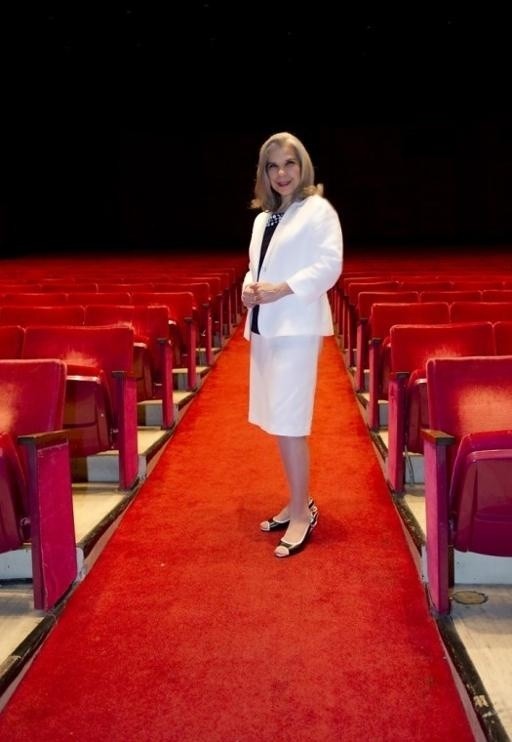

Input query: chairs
[0,256,243,610]
[327,256,512,616]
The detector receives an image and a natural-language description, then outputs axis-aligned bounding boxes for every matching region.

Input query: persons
[239,131,345,560]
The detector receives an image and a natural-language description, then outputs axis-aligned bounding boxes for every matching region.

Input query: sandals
[261,495,317,531]
[275,510,317,557]
